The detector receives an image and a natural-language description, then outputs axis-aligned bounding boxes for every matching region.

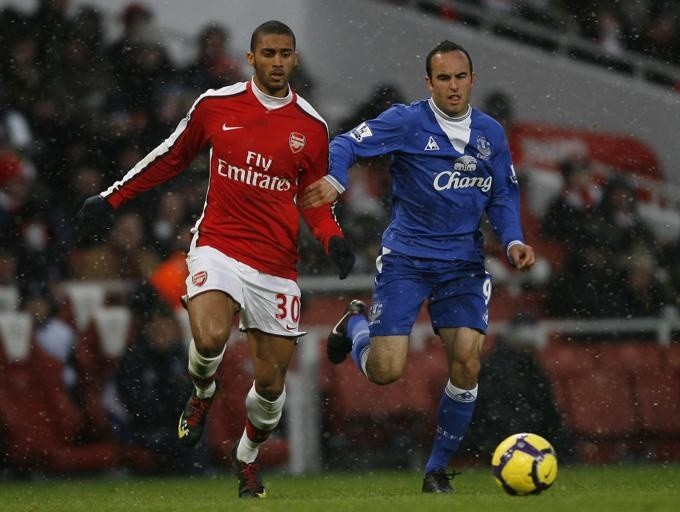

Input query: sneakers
[230,438,268,500]
[175,376,220,449]
[325,295,369,364]
[421,469,463,496]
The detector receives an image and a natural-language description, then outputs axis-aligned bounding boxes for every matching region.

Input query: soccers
[492,434,558,495]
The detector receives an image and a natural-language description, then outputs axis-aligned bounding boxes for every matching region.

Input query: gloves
[326,236,356,280]
[74,195,114,239]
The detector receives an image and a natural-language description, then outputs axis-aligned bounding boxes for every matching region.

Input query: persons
[79,19,354,498]
[301,42,535,494]
